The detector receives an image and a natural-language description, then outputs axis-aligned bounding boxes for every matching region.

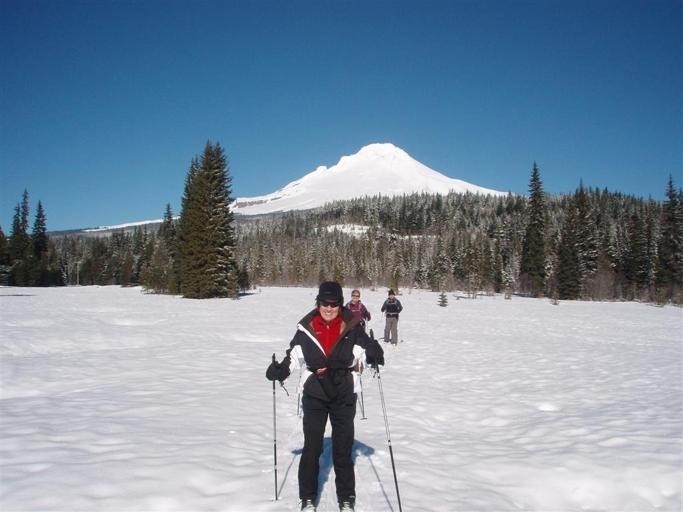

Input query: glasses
[318,299,341,307]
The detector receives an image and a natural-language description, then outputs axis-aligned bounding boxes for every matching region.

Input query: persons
[265,281,385,511]
[344,289,370,332]
[380,288,403,346]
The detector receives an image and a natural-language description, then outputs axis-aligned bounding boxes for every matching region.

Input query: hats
[389,289,395,296]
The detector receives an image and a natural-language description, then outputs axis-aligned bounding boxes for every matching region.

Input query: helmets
[318,281,342,303]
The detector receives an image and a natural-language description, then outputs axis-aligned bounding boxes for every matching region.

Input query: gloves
[364,311,370,320]
[266,361,289,381]
[366,342,380,363]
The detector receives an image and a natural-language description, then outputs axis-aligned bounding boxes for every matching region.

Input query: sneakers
[302,499,315,512]
[339,497,353,512]
